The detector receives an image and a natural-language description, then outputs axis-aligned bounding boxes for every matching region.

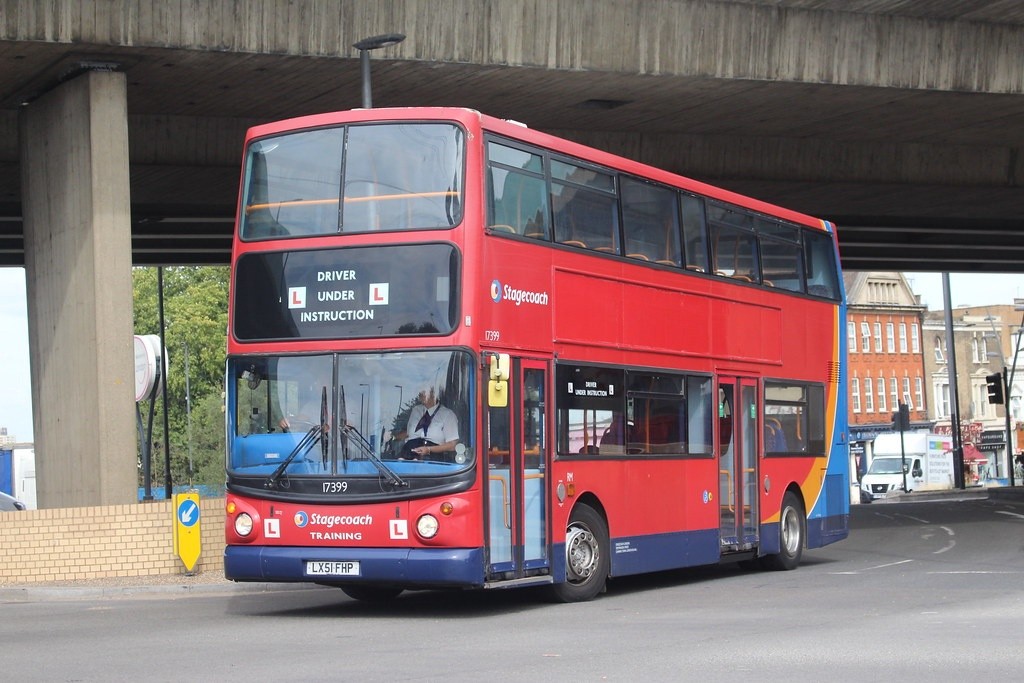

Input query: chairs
[599,412,789,455]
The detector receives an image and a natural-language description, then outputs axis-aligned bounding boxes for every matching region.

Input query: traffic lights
[891,411,901,432]
[986,372,1004,405]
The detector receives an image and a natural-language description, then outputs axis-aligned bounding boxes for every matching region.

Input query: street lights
[359,384,370,442]
[180,341,193,488]
[394,385,403,416]
[351,29,407,455]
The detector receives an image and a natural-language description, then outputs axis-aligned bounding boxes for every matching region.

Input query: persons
[398,381,460,462]
[280,371,333,434]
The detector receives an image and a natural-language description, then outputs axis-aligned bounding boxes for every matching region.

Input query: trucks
[860,433,964,504]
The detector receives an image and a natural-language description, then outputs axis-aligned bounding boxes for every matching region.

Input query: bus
[224,105,850,605]
